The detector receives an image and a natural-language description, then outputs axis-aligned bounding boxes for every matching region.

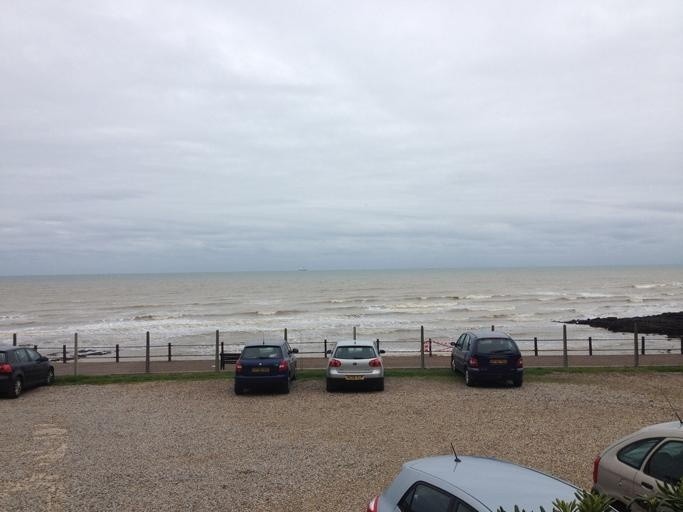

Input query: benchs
[220,353,241,370]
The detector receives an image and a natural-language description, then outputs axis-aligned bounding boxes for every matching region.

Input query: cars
[445,331,525,385]
[368,447,621,511]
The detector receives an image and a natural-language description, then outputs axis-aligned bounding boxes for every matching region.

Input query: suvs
[0,346,56,400]
[323,339,387,392]
[589,417,683,511]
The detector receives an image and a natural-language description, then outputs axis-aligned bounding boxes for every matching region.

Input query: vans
[231,340,299,398]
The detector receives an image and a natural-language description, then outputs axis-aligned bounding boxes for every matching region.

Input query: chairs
[650,452,673,474]
